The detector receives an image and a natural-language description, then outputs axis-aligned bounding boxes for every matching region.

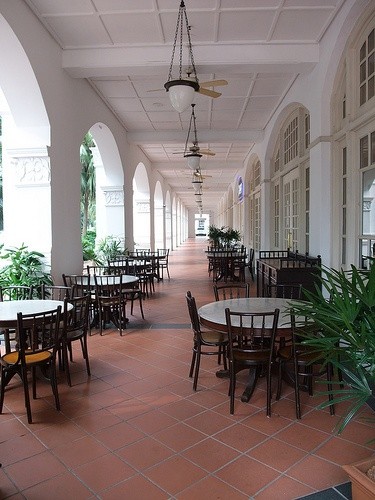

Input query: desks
[205,248,237,253]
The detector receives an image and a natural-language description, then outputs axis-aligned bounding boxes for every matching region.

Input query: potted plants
[280,246,375,500]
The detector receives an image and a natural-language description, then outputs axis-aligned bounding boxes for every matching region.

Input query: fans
[146,69,228,216]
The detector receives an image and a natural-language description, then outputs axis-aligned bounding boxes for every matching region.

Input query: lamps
[190,170,203,192]
[183,104,203,170]
[164,1,199,113]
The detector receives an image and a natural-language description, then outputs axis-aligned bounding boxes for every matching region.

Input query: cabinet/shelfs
[256,248,321,302]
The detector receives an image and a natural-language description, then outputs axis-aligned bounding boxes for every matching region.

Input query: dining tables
[129,253,166,280]
[105,261,151,295]
[198,298,318,403]
[207,253,247,281]
[74,274,139,330]
[0,300,74,387]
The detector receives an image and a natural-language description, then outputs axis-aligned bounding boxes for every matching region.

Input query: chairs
[0,243,344,424]
